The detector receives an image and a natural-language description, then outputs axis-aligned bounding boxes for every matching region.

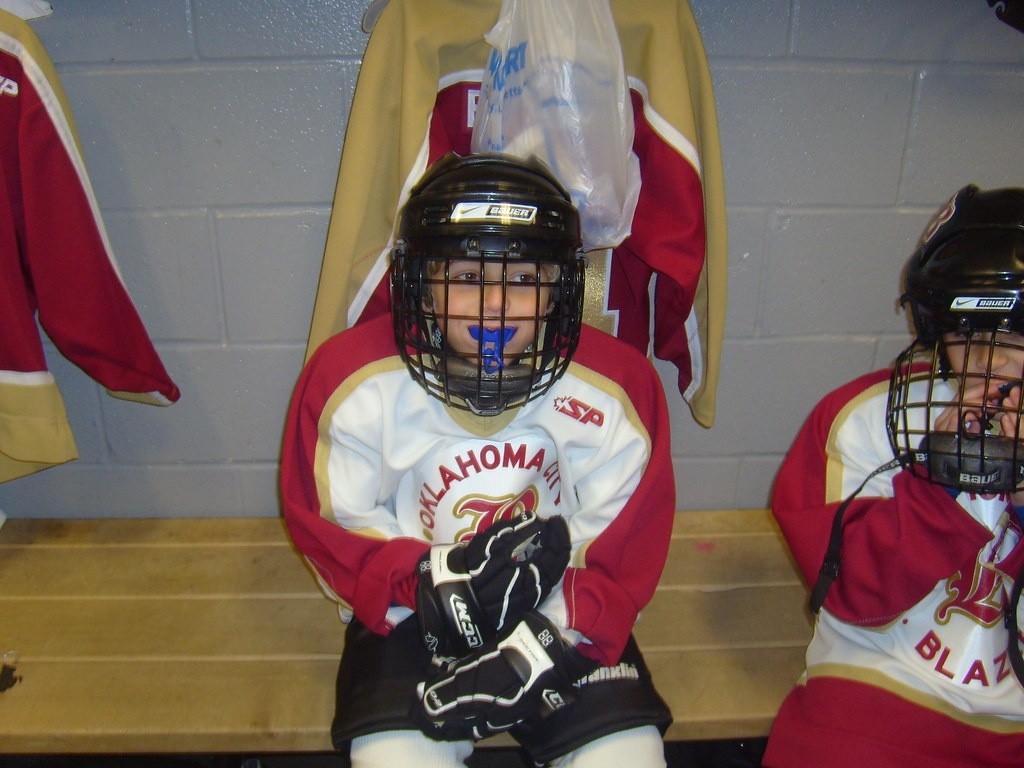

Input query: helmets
[389,151,587,416]
[886,184,1024,492]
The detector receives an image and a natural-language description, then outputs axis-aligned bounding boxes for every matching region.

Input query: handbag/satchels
[473,1,642,251]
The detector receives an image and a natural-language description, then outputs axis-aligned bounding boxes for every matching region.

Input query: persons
[761,183,1024,768]
[280,150,675,768]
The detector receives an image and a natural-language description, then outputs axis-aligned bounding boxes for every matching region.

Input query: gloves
[414,511,572,655]
[410,609,596,740]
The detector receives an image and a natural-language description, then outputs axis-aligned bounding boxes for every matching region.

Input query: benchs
[0,507,815,754]
